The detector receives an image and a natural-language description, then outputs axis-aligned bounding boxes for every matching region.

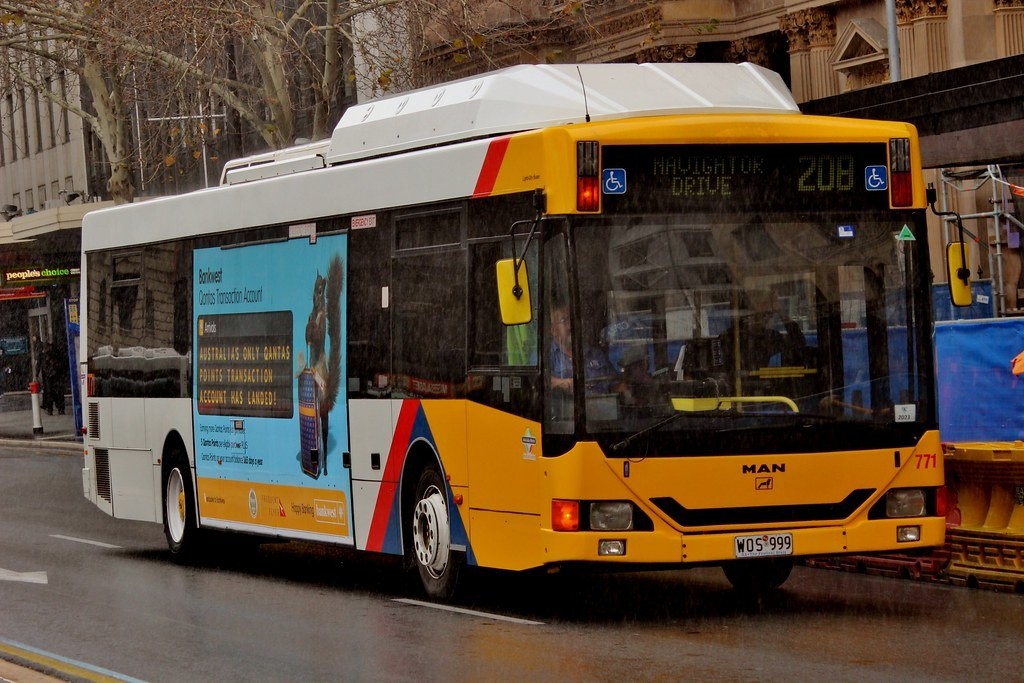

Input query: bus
[75,61,974,608]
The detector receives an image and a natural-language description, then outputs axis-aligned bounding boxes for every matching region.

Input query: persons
[719,289,808,386]
[30,334,67,415]
[531,298,634,418]
